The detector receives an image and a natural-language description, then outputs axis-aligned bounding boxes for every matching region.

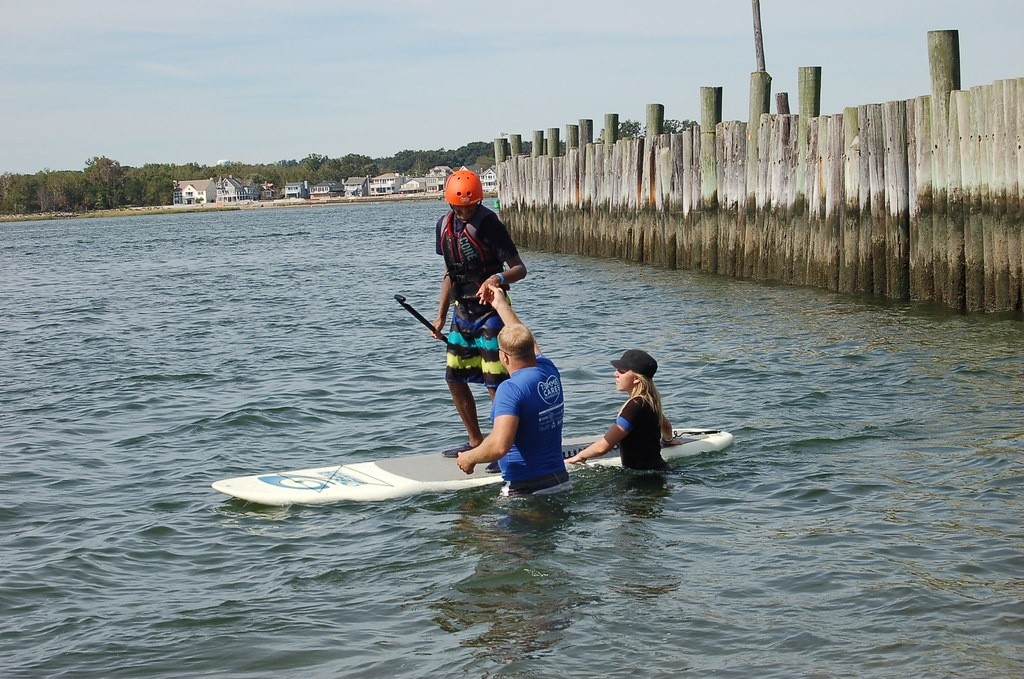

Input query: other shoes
[442,440,475,457]
[485,461,501,473]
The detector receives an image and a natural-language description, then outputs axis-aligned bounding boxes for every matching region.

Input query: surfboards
[211,428,733,507]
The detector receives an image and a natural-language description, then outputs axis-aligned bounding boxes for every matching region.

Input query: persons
[564,349,684,474]
[431,169,528,473]
[456,282,571,500]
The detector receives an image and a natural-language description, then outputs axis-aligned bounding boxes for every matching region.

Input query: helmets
[445,170,483,206]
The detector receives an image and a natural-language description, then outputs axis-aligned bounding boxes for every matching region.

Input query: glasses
[617,366,631,374]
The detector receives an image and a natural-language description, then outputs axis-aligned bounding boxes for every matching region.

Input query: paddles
[393,293,448,344]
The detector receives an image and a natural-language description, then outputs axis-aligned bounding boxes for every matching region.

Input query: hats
[610,348,658,378]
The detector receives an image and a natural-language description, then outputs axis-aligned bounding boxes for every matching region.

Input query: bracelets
[496,273,505,284]
